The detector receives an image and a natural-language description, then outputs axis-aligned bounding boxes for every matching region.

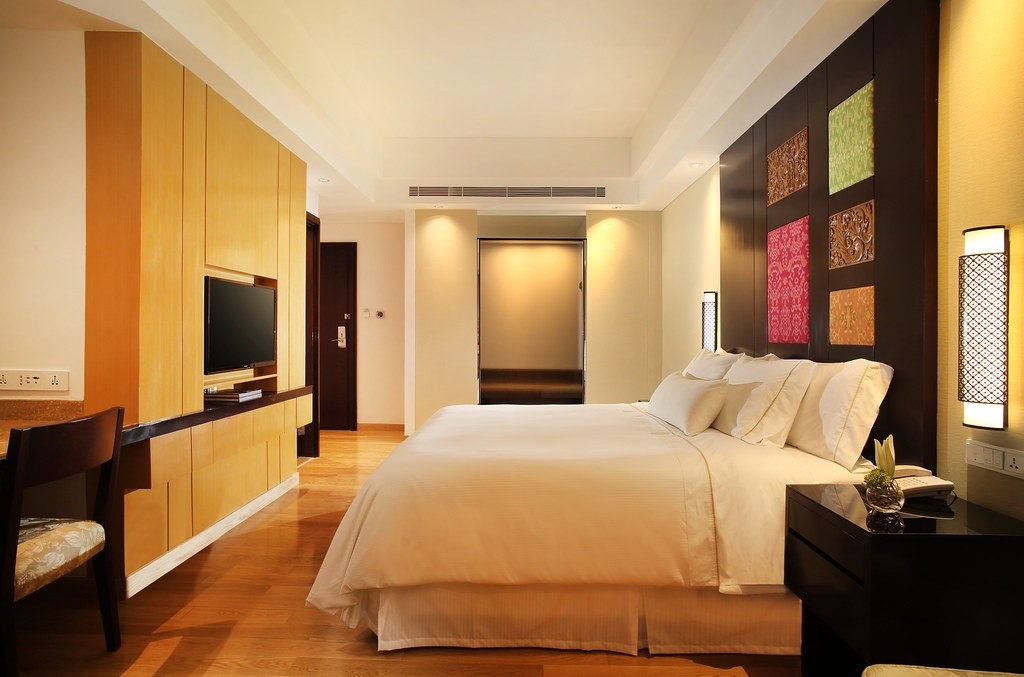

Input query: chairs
[0,405,130,677]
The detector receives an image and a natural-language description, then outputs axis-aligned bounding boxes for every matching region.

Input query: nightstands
[782,479,1024,677]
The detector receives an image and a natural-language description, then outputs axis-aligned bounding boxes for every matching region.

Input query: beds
[301,405,876,657]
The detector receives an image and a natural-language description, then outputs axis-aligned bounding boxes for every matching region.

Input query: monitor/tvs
[202,275,277,374]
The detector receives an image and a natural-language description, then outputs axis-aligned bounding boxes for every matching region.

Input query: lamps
[700,291,718,352]
[957,225,1010,430]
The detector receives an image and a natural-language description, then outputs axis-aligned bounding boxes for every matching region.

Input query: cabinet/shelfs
[84,30,314,598]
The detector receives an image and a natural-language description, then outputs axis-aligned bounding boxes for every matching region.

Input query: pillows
[709,355,819,449]
[786,359,895,474]
[644,370,731,437]
[681,348,743,382]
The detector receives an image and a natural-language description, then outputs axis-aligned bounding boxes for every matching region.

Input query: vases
[865,483,905,513]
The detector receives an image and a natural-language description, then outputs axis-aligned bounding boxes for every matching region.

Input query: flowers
[864,434,902,511]
